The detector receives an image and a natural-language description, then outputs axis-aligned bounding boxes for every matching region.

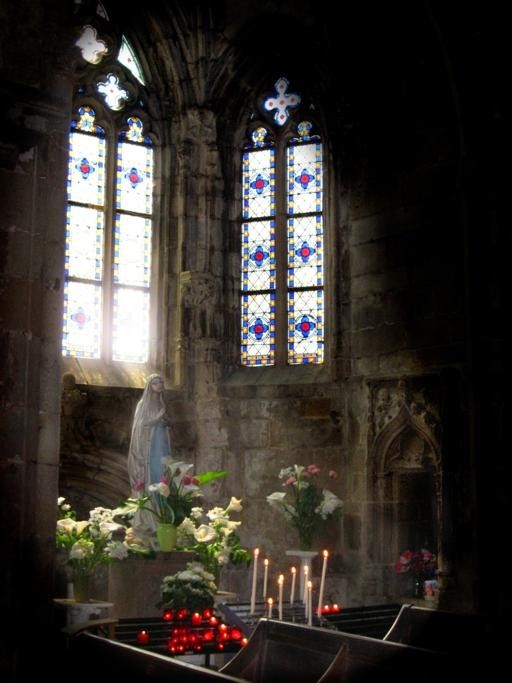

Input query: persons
[61,368,92,451]
[126,371,174,536]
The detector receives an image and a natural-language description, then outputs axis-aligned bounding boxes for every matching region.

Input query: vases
[286,550,319,601]
[157,524,176,550]
[72,573,95,603]
[410,577,425,598]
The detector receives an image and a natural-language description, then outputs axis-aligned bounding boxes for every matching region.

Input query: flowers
[117,456,227,525]
[265,464,344,550]
[177,495,251,568]
[394,548,437,594]
[155,562,220,612]
[55,495,150,576]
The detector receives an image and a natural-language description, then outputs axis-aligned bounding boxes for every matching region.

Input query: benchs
[216,617,437,682]
[381,603,479,651]
[73,628,254,683]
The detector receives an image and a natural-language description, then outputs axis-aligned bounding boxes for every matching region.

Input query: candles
[137,547,339,654]
